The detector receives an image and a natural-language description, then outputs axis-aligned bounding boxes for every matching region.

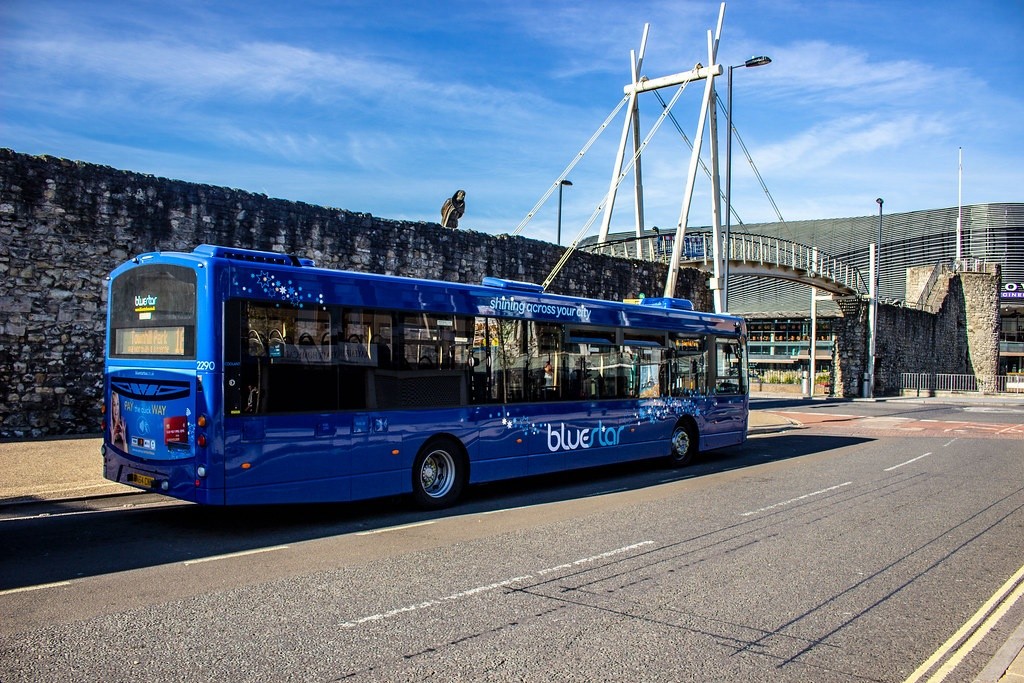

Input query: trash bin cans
[801,371,810,396]
[863,372,870,398]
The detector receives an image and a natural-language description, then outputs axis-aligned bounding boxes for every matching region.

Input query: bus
[101,244,751,512]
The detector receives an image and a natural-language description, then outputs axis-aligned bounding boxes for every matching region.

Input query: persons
[440,189,465,228]
[542,363,554,389]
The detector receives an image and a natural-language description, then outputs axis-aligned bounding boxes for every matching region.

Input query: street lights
[870,197,884,399]
[652,226,660,262]
[722,56,772,312]
[558,180,573,246]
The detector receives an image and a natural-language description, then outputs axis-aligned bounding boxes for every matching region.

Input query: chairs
[248,329,391,363]
